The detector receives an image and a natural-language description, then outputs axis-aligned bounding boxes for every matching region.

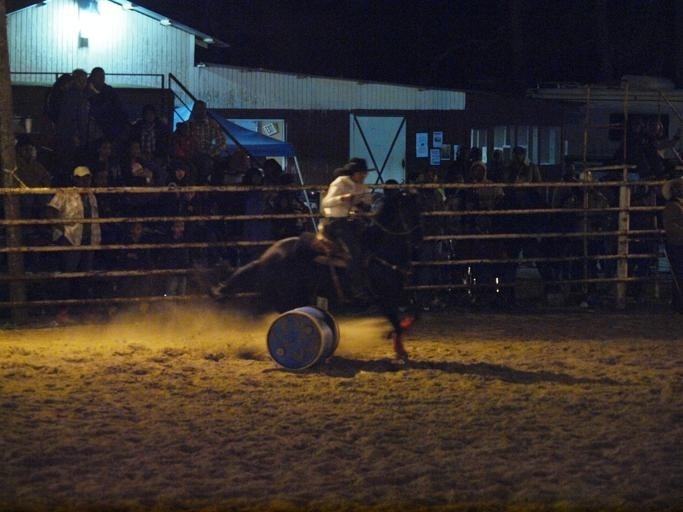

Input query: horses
[207,189,433,369]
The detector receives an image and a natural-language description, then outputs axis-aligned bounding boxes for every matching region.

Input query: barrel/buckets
[266,306,340,373]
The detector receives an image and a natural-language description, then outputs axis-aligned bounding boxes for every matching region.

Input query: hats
[74,166,92,177]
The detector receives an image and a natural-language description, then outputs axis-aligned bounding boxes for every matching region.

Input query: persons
[319,157,374,276]
[631,120,670,178]
[40,63,228,141]
[0,120,314,324]
[384,146,683,313]
